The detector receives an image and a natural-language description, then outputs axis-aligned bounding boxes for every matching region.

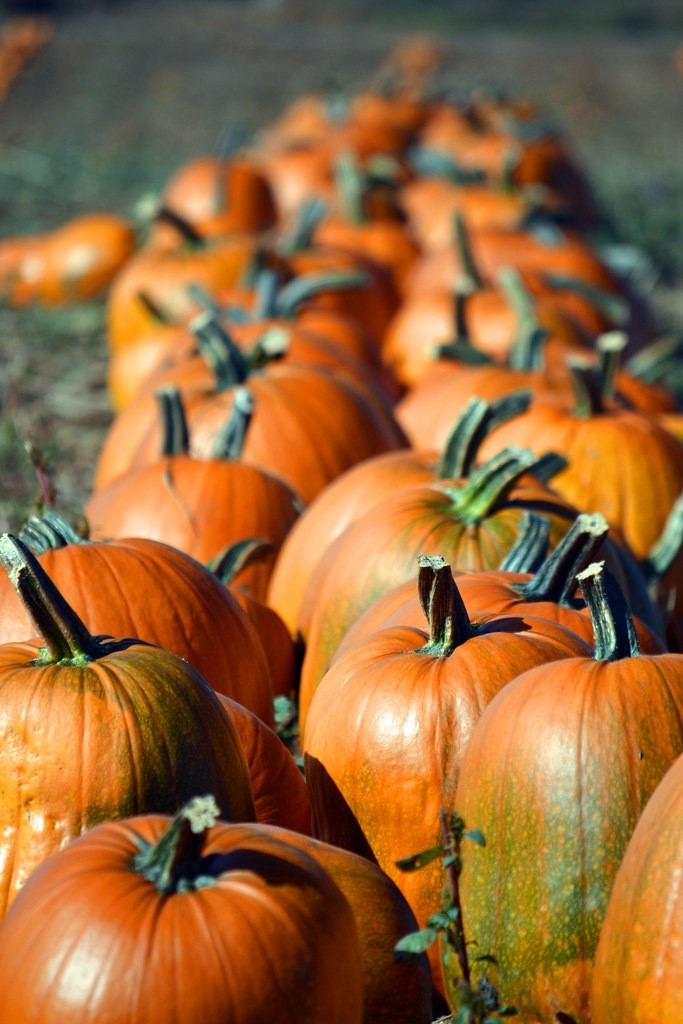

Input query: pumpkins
[0,20,683,1024]
